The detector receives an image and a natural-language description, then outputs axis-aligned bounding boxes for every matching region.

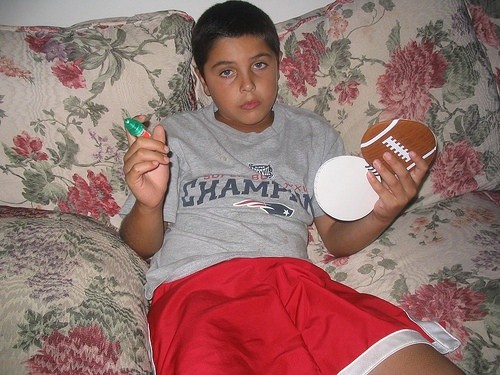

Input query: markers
[124,117,153,140]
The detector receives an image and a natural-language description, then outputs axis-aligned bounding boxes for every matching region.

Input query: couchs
[0,0,500,374]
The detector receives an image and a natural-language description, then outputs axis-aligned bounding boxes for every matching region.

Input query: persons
[118,0,465,375]
